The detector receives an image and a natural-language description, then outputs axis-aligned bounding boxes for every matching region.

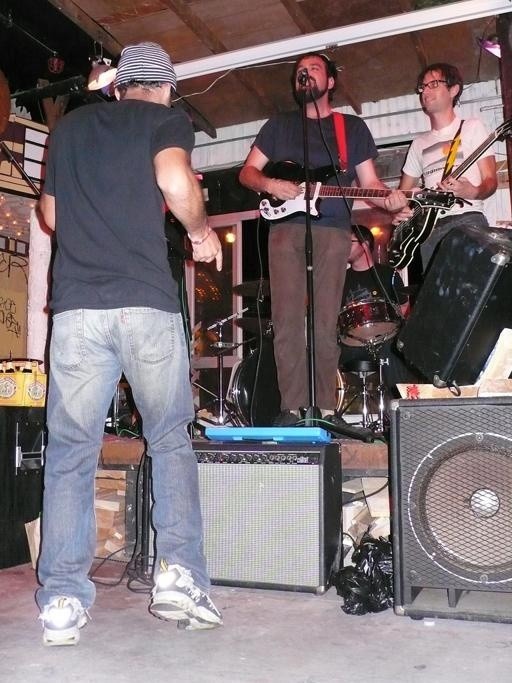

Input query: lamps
[87,40,117,90]
[225,226,236,243]
[476,35,502,62]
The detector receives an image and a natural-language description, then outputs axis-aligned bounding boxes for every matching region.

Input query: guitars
[259,159,456,223]
[386,118,512,270]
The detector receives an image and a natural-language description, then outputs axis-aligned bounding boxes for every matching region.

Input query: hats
[113,42,180,92]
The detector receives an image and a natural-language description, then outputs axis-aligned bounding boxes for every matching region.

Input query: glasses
[351,238,366,244]
[415,79,450,95]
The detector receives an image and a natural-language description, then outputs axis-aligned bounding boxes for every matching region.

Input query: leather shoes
[320,414,374,439]
[272,409,304,428]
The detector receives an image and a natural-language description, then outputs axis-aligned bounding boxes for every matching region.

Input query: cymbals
[233,317,275,338]
[232,280,271,297]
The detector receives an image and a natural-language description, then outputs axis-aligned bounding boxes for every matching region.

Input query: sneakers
[40,598,88,645]
[148,564,225,633]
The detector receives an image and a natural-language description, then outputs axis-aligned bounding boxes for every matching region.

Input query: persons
[396,63,498,275]
[338,224,408,421]
[36,40,223,648]
[241,54,406,437]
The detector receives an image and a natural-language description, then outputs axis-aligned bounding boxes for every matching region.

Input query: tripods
[277,86,376,445]
[195,327,241,427]
[339,372,391,432]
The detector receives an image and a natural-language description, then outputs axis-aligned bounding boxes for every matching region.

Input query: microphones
[298,68,309,85]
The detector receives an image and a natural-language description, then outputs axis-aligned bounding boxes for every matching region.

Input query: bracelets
[265,178,276,194]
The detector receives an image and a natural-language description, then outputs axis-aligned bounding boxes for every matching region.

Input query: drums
[334,367,364,414]
[337,298,405,347]
[230,346,281,427]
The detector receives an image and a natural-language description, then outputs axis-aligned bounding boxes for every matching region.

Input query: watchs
[188,228,211,246]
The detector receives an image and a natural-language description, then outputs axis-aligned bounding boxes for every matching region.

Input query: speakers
[386,396,512,617]
